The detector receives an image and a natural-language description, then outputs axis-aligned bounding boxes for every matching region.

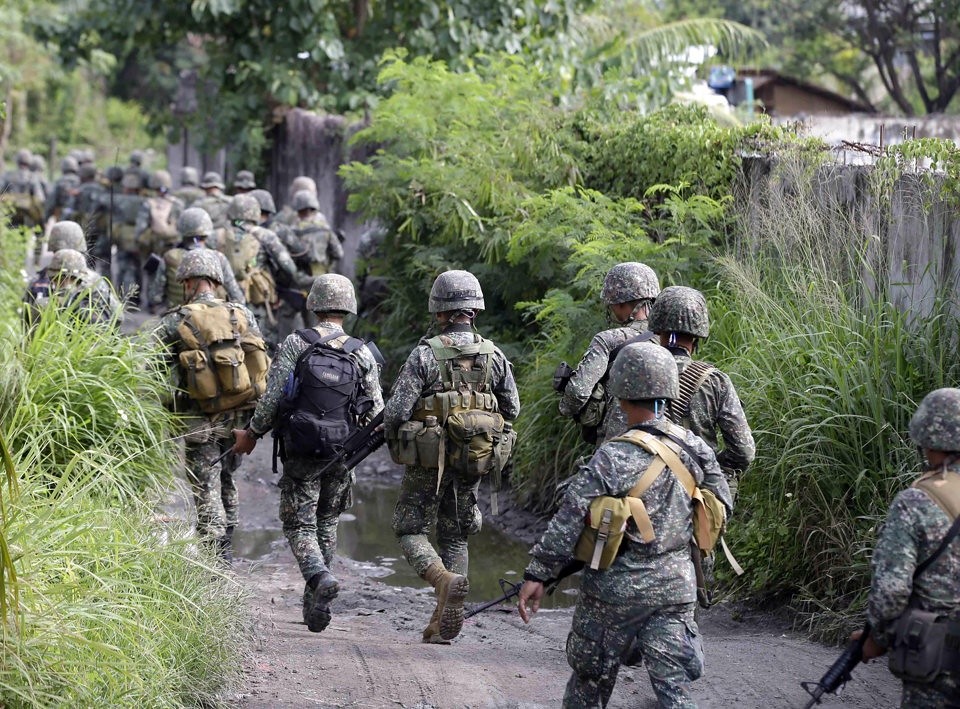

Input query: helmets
[600,262,661,304]
[306,274,358,315]
[18,147,320,250]
[648,286,708,337]
[428,270,485,313]
[609,342,680,400]
[176,249,224,285]
[47,249,87,280]
[909,387,960,452]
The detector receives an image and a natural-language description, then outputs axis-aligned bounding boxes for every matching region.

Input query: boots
[307,572,340,632]
[423,605,452,644]
[220,526,235,566]
[425,562,469,640]
[303,584,312,625]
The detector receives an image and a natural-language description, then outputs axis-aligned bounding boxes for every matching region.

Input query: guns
[799,633,870,709]
[309,410,391,485]
[553,360,574,393]
[462,557,587,620]
[207,399,292,468]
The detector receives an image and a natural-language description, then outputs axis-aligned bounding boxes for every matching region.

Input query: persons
[231,274,385,634]
[0,150,344,544]
[560,261,757,680]
[518,342,734,709]
[384,269,521,644]
[850,387,960,709]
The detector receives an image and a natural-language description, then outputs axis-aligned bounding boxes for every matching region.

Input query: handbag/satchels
[447,410,504,475]
[888,608,945,682]
[694,489,726,550]
[575,494,655,569]
[183,408,212,443]
[245,269,278,305]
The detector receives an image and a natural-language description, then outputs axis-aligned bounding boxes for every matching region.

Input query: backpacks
[274,328,374,458]
[177,300,272,413]
